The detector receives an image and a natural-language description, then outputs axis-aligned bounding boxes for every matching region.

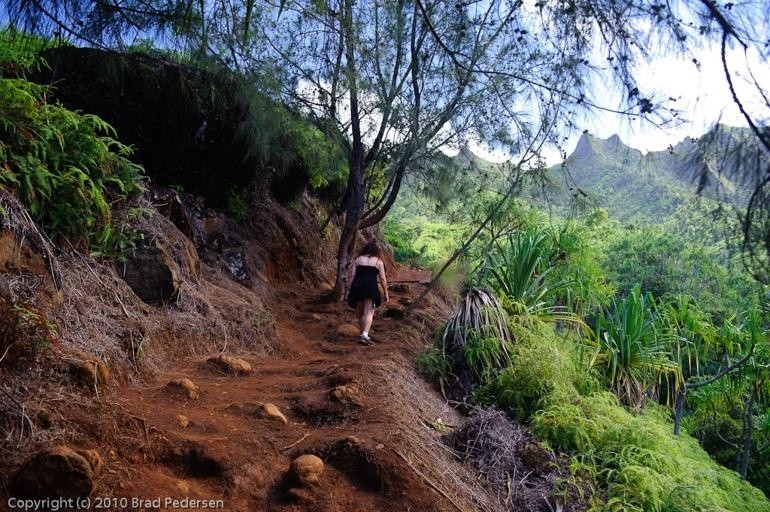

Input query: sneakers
[359,335,375,345]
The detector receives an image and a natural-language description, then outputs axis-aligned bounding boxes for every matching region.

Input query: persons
[345,238,390,346]
